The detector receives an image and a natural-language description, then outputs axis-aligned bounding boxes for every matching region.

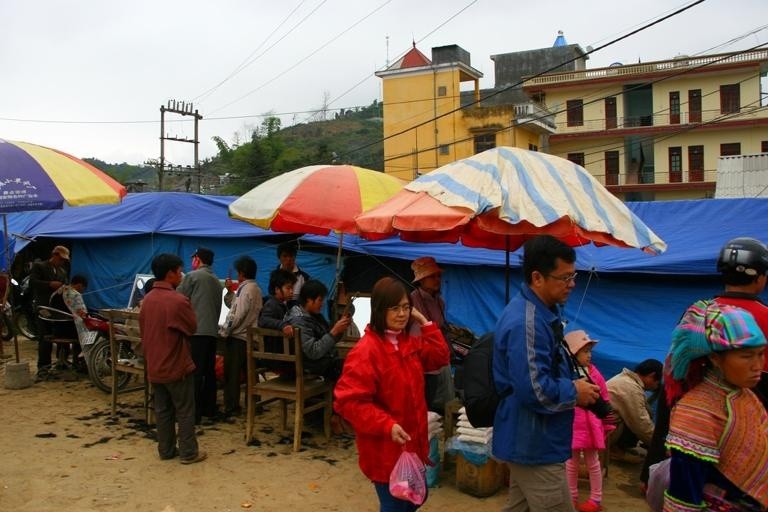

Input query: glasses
[386,305,410,311]
[550,273,578,282]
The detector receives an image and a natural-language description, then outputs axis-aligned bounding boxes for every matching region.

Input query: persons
[492,236,600,511]
[639,236,768,497]
[333,277,452,512]
[129,243,352,464]
[49,274,88,339]
[29,245,71,379]
[564,330,618,512]
[664,300,768,511]
[407,256,445,411]
[605,359,664,464]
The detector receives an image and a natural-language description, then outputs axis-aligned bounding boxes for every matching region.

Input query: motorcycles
[14,277,44,340]
[0,271,14,340]
[83,279,146,392]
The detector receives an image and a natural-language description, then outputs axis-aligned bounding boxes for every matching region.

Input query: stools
[444,397,463,471]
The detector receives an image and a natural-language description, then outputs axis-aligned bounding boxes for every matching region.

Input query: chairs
[108,309,155,427]
[37,305,79,381]
[246,327,332,452]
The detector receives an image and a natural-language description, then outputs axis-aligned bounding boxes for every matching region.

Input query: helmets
[717,237,768,275]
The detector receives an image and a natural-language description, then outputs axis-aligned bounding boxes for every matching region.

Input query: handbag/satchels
[462,331,510,428]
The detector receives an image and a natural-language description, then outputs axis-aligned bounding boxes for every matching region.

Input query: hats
[53,245,71,262]
[411,256,444,283]
[192,248,214,259]
[564,330,599,356]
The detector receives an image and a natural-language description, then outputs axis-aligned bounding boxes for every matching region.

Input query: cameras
[577,374,612,418]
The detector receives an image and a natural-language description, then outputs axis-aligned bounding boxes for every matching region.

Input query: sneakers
[180,452,207,464]
[329,414,355,439]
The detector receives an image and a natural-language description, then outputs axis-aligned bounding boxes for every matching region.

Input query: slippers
[580,499,603,512]
[610,452,641,464]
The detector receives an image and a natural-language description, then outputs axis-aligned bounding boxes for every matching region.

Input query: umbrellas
[0,139,127,362]
[228,164,407,322]
[352,145,667,305]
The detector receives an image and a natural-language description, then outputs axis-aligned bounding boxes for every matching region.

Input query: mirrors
[343,292,372,341]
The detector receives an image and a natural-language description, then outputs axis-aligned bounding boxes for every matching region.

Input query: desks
[334,340,355,360]
